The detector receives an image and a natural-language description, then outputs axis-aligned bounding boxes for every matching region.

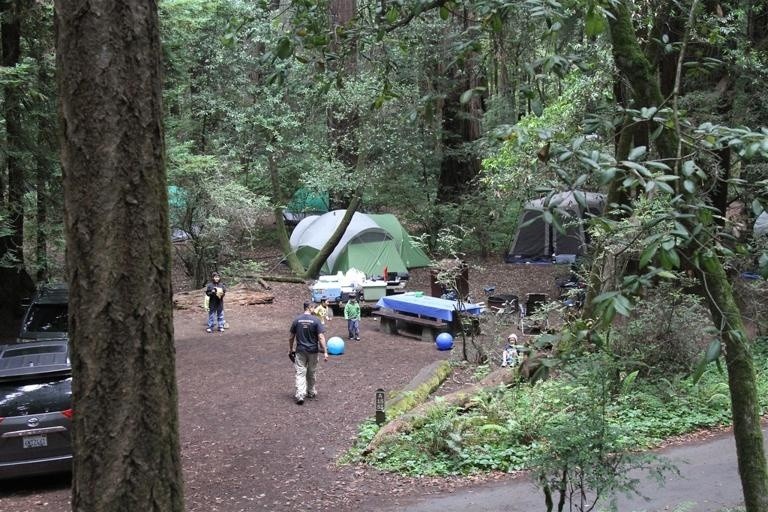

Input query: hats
[507,334,517,344]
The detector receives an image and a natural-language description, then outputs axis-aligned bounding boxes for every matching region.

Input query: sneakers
[308,391,317,398]
[218,328,224,332]
[297,398,304,404]
[206,328,211,332]
[350,337,360,340]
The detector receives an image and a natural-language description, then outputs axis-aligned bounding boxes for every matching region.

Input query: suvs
[1,342,77,485]
[16,283,76,342]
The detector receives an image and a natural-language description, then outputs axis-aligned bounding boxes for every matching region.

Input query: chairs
[517,294,549,336]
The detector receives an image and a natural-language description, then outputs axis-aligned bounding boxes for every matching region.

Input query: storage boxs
[363,283,388,302]
[314,288,362,303]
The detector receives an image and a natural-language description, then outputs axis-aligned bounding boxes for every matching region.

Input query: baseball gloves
[289,351,295,361]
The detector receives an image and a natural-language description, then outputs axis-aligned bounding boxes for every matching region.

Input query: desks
[376,290,481,322]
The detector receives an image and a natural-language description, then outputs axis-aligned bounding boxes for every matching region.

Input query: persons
[501,333,519,367]
[344,293,361,340]
[288,300,328,404]
[205,283,228,328]
[205,272,226,333]
[313,296,332,352]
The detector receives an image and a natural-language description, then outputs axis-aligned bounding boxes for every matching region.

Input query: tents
[169,184,199,242]
[282,210,436,282]
[277,183,328,226]
[506,190,610,264]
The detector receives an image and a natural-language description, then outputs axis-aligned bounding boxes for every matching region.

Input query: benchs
[371,309,447,342]
